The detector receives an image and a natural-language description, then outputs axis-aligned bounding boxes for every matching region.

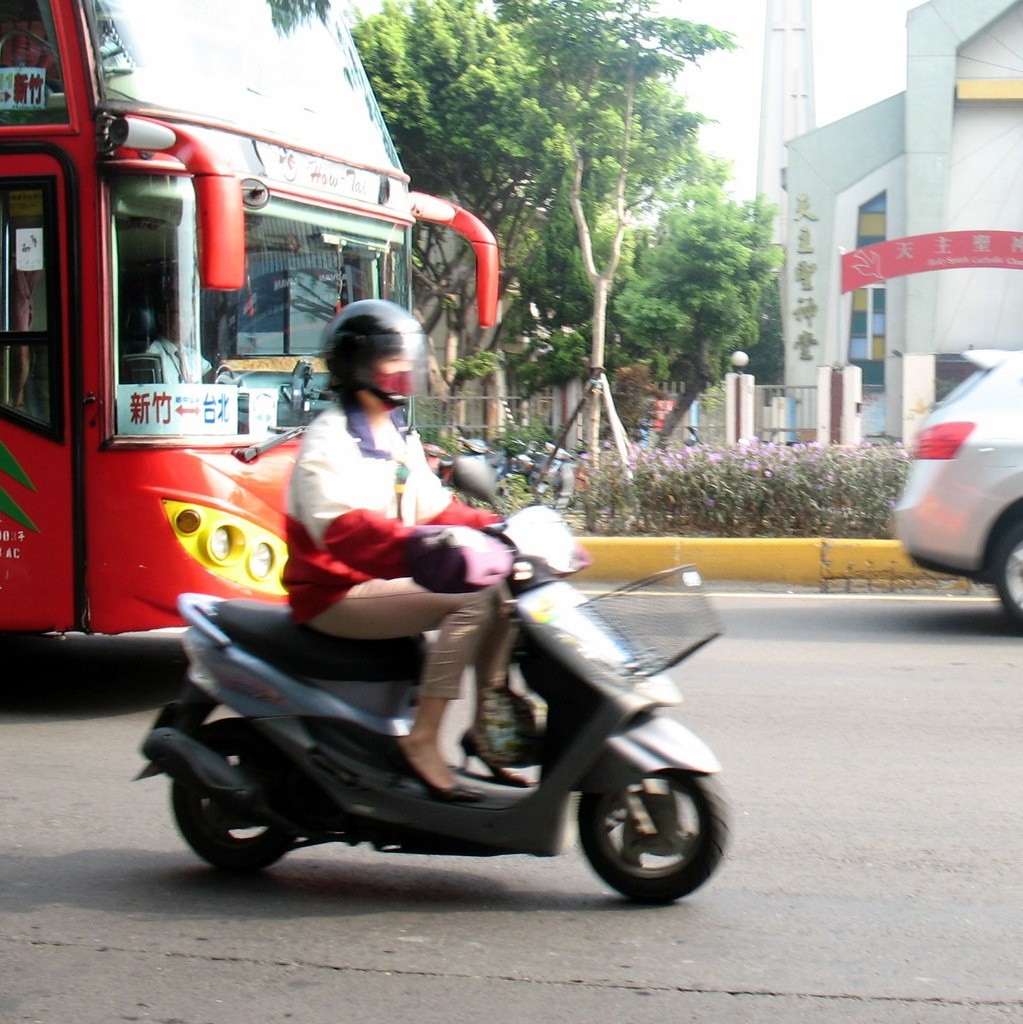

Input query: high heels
[461,730,537,788]
[391,736,485,801]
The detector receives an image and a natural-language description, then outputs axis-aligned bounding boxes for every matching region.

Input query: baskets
[579,564,727,677]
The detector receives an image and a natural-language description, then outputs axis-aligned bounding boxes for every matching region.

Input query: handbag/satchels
[478,685,546,768]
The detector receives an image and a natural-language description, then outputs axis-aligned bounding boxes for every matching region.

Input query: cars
[887,350,1023,646]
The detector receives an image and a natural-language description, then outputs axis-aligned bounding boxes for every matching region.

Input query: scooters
[129,453,731,909]
[416,422,704,510]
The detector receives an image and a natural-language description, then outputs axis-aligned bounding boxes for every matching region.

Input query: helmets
[326,298,421,407]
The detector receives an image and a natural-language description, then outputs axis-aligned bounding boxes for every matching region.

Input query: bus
[2,0,501,650]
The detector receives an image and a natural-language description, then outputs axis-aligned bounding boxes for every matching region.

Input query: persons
[147,286,216,384]
[279,298,532,802]
